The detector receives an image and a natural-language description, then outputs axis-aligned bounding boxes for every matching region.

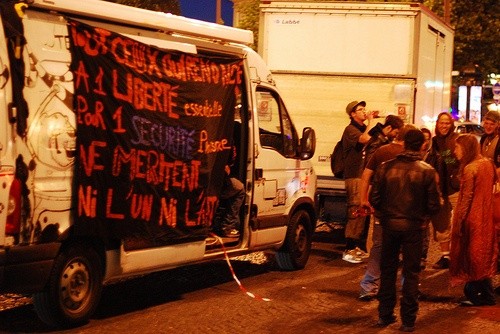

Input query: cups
[440,239,450,255]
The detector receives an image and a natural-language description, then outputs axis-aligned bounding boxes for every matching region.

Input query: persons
[451,134,498,305]
[359,124,417,300]
[368,129,445,332]
[216,121,245,237]
[342,101,372,264]
[362,115,444,271]
[427,111,463,268]
[480,110,500,274]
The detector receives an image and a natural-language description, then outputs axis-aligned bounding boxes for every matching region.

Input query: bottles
[364,110,388,118]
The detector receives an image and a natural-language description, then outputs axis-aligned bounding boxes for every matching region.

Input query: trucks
[0,0,320,333]
[257,0,457,255]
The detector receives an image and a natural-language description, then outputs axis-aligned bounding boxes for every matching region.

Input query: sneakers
[432,256,450,269]
[398,322,415,332]
[342,246,370,264]
[376,313,399,325]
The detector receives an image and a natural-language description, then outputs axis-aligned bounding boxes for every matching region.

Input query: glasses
[355,108,365,112]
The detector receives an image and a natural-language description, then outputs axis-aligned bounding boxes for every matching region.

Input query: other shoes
[460,289,496,307]
[360,283,376,299]
[225,228,241,237]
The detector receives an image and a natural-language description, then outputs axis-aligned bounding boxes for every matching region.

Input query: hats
[484,110,500,122]
[346,101,366,115]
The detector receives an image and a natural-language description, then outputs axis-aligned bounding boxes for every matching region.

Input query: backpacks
[329,140,345,180]
